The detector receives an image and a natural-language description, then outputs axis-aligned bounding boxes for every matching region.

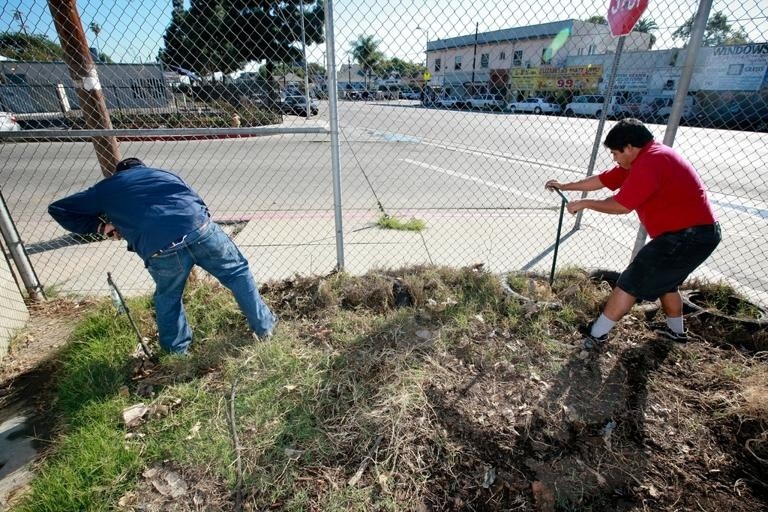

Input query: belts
[151,235,186,255]
[672,222,720,236]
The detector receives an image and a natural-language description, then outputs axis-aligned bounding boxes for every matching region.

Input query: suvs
[467,94,504,110]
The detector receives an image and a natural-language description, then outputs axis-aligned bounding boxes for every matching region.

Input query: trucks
[640,89,767,131]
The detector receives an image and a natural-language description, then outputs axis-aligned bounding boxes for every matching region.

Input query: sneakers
[651,322,691,344]
[577,318,610,343]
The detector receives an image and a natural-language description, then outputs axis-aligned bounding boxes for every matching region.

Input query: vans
[563,95,626,119]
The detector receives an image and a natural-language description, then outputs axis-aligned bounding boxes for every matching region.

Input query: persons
[46,156,280,356]
[543,118,722,347]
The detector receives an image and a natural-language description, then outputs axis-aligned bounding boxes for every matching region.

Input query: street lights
[416,26,430,105]
[14,9,36,63]
[90,21,102,63]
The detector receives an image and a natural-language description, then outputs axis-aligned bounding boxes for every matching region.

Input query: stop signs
[607,0,649,37]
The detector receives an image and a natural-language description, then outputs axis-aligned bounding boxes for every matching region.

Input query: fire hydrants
[230,112,241,129]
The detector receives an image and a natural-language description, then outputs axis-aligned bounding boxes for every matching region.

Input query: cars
[248,82,319,117]
[506,98,561,115]
[0,112,21,132]
[347,82,421,101]
[436,95,464,108]
